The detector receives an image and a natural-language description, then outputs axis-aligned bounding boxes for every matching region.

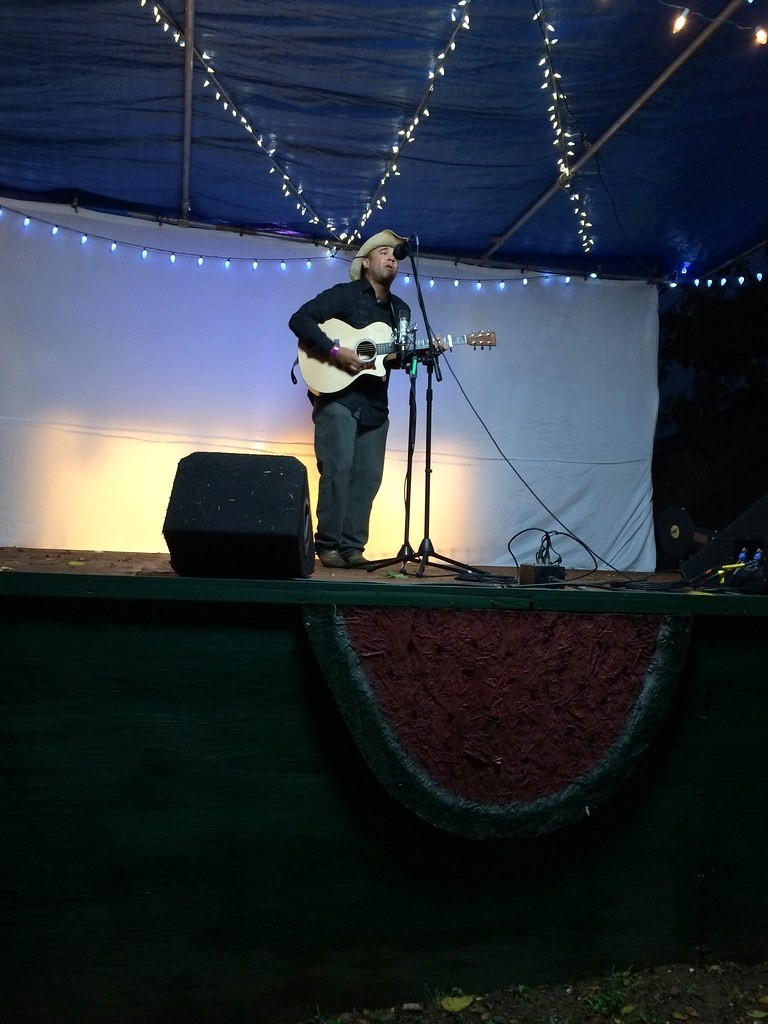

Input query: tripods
[344,247,493,577]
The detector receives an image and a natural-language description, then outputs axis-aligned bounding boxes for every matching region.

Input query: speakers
[162,452,316,579]
[680,493,768,597]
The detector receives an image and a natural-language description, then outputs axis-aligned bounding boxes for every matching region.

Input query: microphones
[398,309,412,359]
[393,233,416,261]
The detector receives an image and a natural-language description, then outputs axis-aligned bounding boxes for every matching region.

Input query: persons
[288,228,440,568]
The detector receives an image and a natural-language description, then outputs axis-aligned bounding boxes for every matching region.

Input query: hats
[348,229,408,281]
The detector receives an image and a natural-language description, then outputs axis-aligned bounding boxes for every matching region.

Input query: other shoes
[318,550,369,569]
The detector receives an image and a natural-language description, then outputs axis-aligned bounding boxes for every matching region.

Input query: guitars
[297,318,497,397]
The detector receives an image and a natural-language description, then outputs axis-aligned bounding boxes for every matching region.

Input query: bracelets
[331,343,340,357]
